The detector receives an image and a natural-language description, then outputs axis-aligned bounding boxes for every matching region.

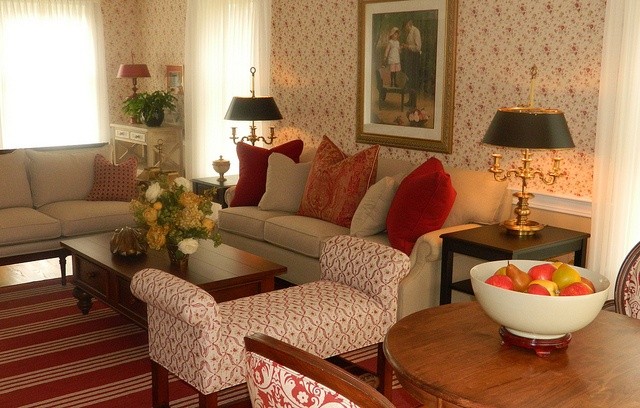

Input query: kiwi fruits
[551,264,581,289]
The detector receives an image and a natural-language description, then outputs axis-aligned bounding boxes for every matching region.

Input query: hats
[388,26,401,39]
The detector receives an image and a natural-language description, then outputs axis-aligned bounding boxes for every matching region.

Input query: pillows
[231,140,302,206]
[350,175,394,237]
[88,155,137,202]
[298,135,379,227]
[388,157,456,255]
[259,151,310,211]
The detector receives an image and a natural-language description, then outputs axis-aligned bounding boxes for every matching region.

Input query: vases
[166,245,187,265]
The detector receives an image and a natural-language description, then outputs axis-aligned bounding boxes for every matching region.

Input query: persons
[401,19,423,109]
[382,27,403,87]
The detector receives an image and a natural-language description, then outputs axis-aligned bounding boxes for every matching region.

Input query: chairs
[612,243,639,313]
[243,333,391,408]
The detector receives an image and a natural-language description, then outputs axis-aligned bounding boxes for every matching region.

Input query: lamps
[224,66,285,145]
[479,63,576,237]
[117,47,151,124]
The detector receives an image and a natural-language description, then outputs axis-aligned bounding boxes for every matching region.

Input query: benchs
[120,236,410,408]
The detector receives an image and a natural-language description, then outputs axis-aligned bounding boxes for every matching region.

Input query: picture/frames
[165,63,185,95]
[355,0,458,155]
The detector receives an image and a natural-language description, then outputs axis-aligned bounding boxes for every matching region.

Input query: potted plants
[122,91,179,127]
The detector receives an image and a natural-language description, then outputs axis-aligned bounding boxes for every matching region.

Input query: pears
[529,280,559,297]
[506,260,531,291]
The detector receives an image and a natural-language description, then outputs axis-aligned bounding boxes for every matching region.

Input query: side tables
[191,175,238,208]
[436,220,592,306]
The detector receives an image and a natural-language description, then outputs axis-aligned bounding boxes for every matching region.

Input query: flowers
[132,178,224,254]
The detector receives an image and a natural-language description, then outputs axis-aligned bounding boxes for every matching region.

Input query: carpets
[1,274,424,408]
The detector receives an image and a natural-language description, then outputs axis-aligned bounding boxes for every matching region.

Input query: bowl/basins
[469,256,612,342]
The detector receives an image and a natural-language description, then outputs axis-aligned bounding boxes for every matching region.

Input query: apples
[581,277,595,293]
[484,275,513,290]
[528,264,556,281]
[559,282,593,296]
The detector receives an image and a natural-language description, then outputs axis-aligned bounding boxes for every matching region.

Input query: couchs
[214,143,510,321]
[0,141,150,280]
